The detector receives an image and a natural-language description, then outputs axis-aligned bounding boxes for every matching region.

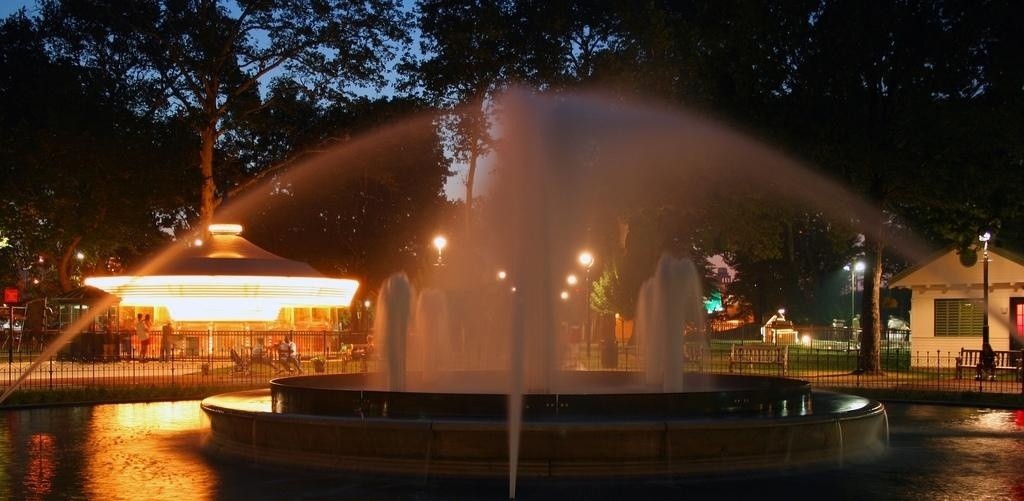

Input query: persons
[976,342,999,382]
[333,334,376,373]
[142,315,151,329]
[229,335,303,376]
[159,323,174,362]
[136,314,151,361]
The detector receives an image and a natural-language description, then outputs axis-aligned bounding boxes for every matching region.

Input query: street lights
[971,231,999,374]
[842,261,867,326]
[365,299,371,332]
[432,236,449,370]
[559,248,594,362]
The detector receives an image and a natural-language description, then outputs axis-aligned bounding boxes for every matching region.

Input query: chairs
[683,342,703,373]
[230,349,301,380]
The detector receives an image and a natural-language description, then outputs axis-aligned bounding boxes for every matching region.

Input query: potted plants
[310,355,326,372]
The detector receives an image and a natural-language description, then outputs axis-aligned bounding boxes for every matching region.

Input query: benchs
[341,343,368,372]
[728,344,789,377]
[955,347,1024,383]
